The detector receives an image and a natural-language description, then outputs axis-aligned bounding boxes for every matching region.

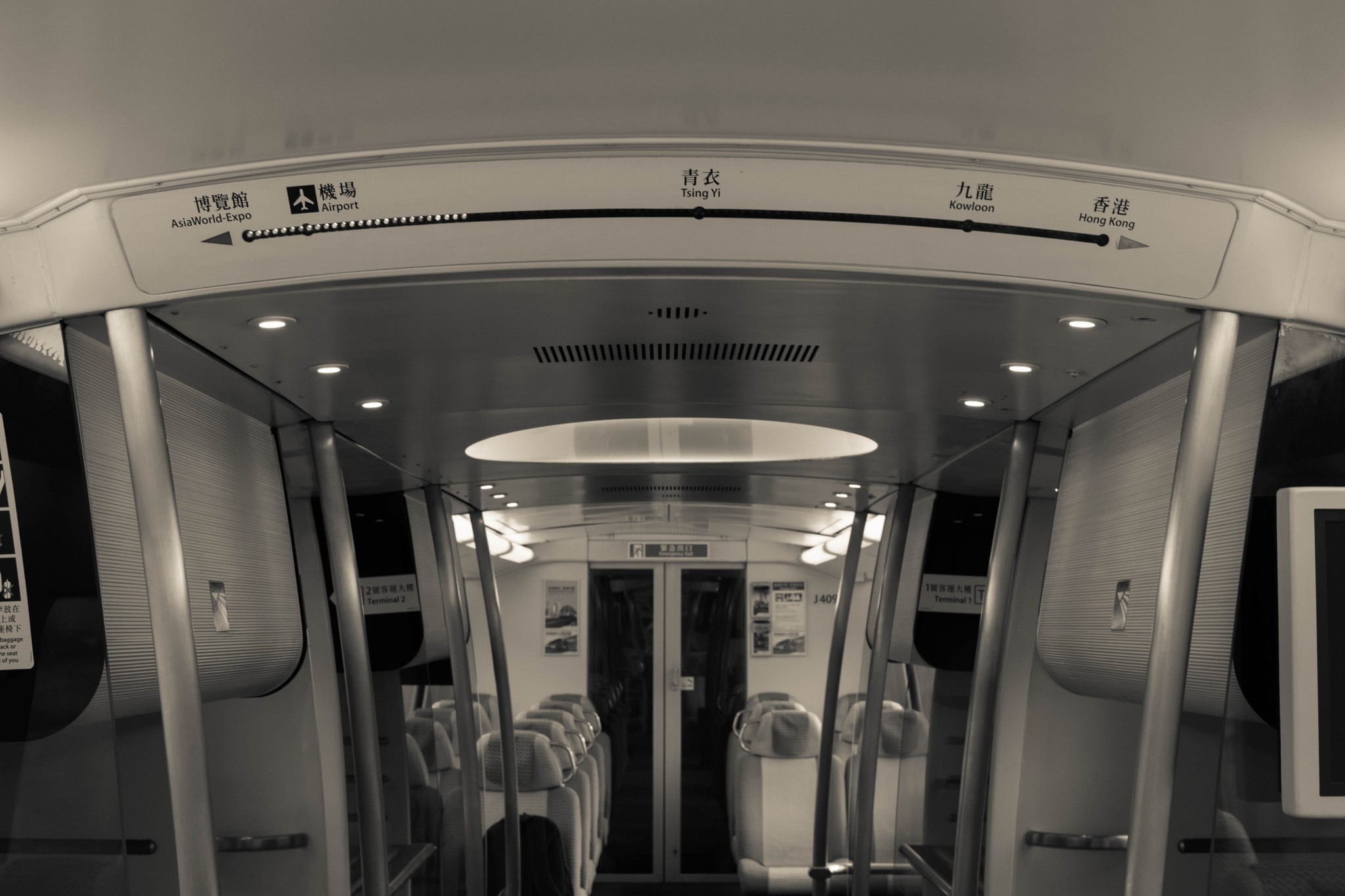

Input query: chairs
[404,693,930,896]
